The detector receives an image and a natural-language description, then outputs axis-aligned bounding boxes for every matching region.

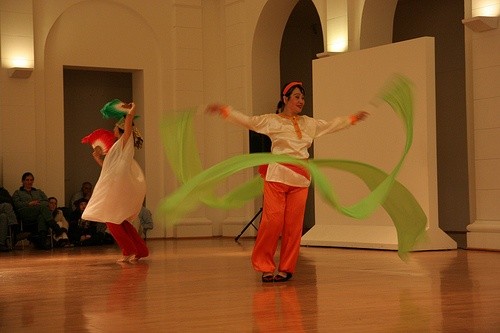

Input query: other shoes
[57,240,74,248]
[132,257,139,261]
[53,226,64,237]
[261,274,273,282]
[118,256,129,261]
[274,273,292,282]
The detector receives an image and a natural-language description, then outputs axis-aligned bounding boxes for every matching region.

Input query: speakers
[249,130,272,154]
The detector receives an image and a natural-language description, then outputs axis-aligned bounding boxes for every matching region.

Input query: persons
[81,102,150,262]
[0,170,154,253]
[204,81,370,282]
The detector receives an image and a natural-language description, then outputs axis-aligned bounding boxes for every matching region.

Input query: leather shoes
[0,246,10,252]
[16,232,31,241]
